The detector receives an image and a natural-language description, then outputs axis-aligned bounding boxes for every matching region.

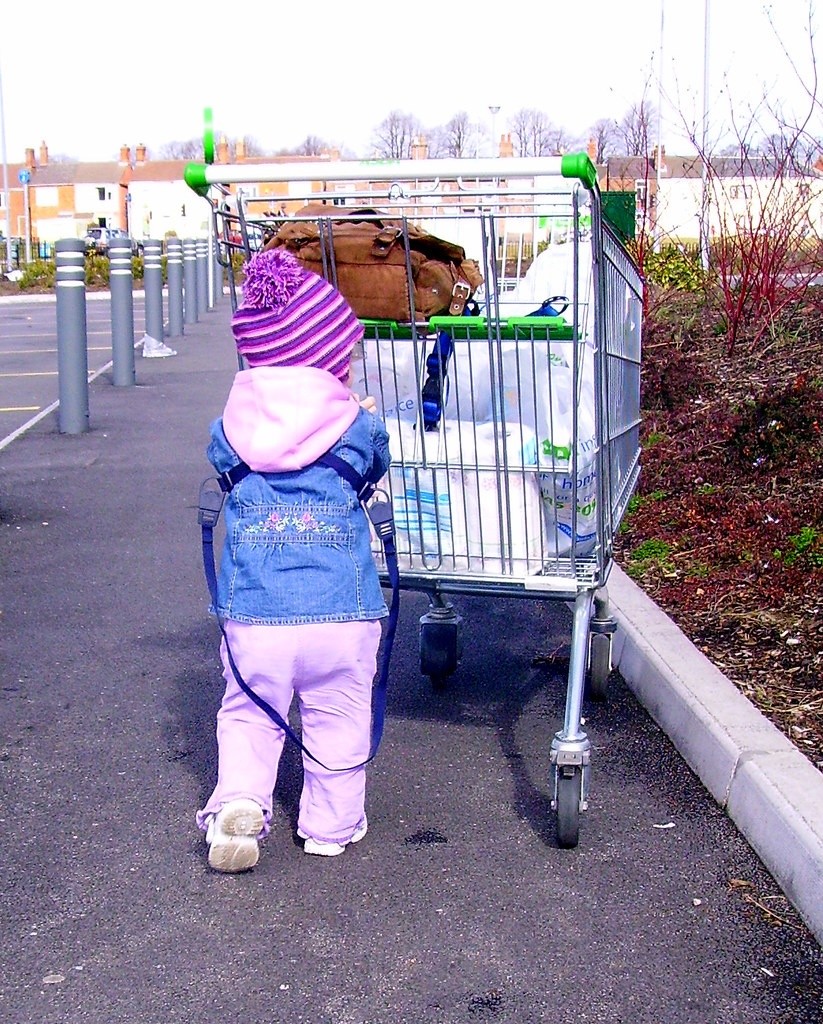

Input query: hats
[230,248,366,386]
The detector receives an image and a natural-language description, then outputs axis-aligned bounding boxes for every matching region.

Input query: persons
[194,249,391,871]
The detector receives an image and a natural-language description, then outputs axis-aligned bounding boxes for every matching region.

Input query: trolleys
[182,153,644,849]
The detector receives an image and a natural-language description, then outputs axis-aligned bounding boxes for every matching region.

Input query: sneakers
[205,799,265,872]
[297,814,368,856]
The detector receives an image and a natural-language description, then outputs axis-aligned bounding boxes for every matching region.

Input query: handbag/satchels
[261,203,485,333]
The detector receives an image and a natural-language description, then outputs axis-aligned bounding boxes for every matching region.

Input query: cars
[217,229,262,253]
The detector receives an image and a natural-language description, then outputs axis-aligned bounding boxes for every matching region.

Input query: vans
[0,237,24,259]
[85,229,144,257]
